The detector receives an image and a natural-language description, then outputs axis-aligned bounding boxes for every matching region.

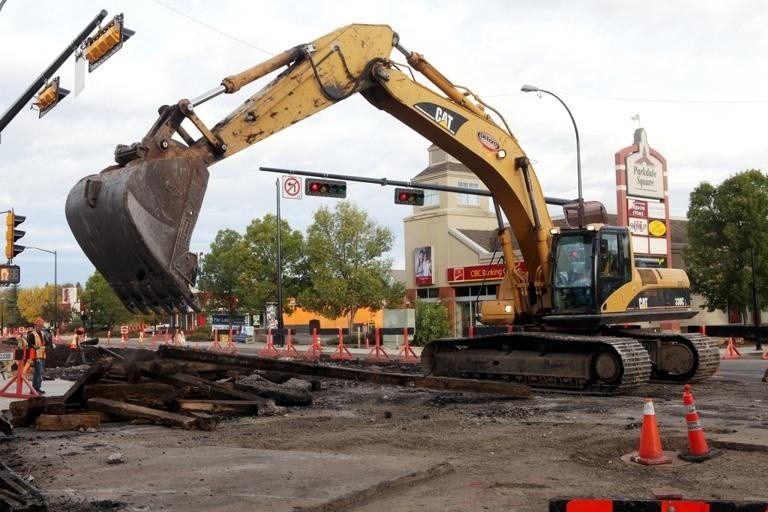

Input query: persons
[177,330,186,345]
[44,328,52,346]
[16,332,28,348]
[28,318,45,395]
[71,328,79,348]
[415,250,432,276]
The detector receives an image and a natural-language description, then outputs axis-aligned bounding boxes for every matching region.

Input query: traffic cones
[677,384,724,463]
[119,334,127,346]
[631,398,672,465]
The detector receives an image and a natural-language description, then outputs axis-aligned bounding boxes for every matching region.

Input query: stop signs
[121,326,129,334]
[19,326,24,332]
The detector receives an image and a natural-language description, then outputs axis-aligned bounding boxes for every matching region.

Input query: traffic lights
[0,264,20,283]
[85,13,135,73]
[5,208,26,264]
[36,76,71,119]
[306,177,346,198]
[394,187,424,205]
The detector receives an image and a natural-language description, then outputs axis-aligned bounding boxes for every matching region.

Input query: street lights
[520,84,584,230]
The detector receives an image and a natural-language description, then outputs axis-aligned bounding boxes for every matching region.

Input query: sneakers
[30,388,45,394]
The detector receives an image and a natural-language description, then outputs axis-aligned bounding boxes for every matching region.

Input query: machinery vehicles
[64,23,720,398]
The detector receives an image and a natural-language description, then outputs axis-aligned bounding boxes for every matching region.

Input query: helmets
[34,318,44,325]
